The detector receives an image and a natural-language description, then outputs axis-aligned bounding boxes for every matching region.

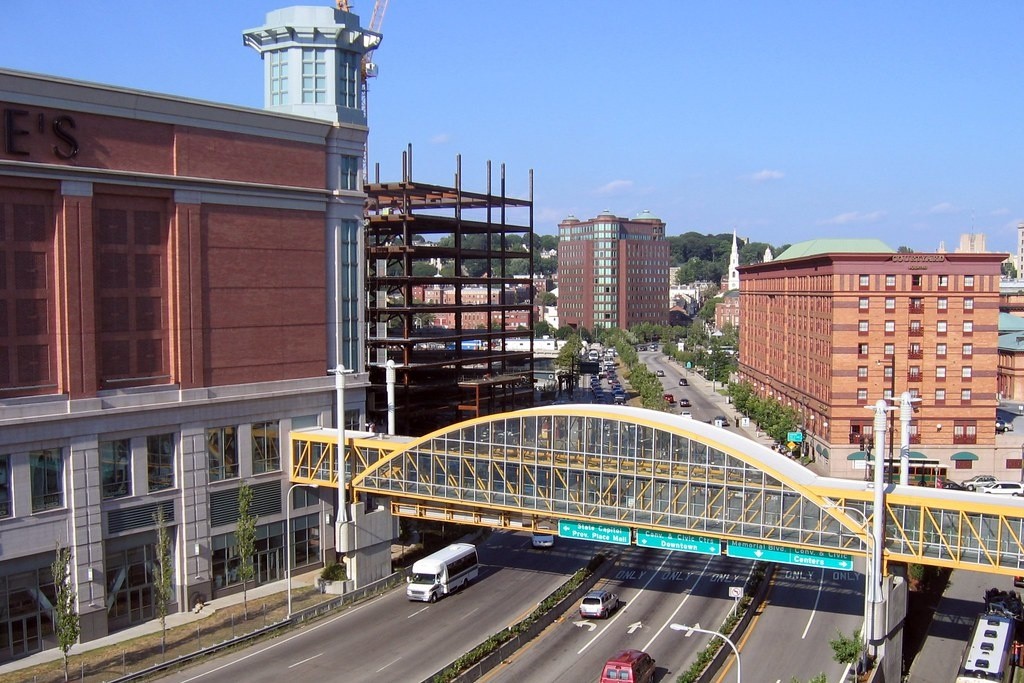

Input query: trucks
[404,541,479,603]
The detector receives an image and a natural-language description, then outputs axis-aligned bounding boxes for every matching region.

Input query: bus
[953,602,1014,683]
[864,457,949,488]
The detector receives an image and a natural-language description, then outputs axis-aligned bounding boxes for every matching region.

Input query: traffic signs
[556,519,632,546]
[635,527,722,557]
[726,539,854,572]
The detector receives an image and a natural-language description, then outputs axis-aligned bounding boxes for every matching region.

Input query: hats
[1012,641,1018,645]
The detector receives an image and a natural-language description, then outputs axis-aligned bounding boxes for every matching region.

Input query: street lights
[822,505,870,674]
[668,623,741,683]
[287,484,319,614]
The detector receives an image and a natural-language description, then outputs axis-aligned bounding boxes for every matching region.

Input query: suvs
[579,588,619,620]
[600,647,657,683]
[980,481,1024,497]
[961,475,999,491]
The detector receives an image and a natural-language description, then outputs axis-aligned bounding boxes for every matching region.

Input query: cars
[942,480,964,490]
[996,419,1014,432]
[1013,556,1024,586]
[715,415,728,426]
[588,342,694,420]
[530,526,555,547]
